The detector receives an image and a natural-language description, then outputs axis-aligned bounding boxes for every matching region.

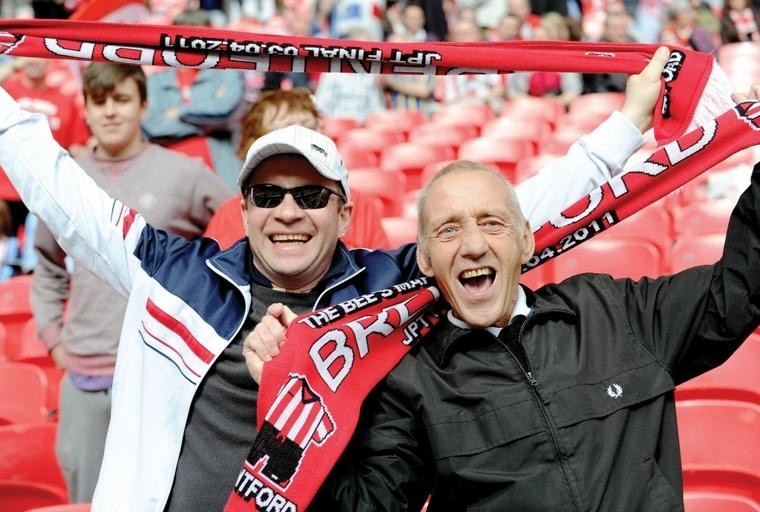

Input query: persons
[0,1,759,112]
[242,153,760,511]
[28,61,224,503]
[0,44,674,511]
[199,93,394,260]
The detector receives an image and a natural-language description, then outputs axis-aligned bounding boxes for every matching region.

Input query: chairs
[0,90,758,512]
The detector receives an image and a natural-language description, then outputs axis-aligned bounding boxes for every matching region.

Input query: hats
[236,124,350,204]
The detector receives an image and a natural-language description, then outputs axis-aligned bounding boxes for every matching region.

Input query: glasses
[242,183,347,209]
